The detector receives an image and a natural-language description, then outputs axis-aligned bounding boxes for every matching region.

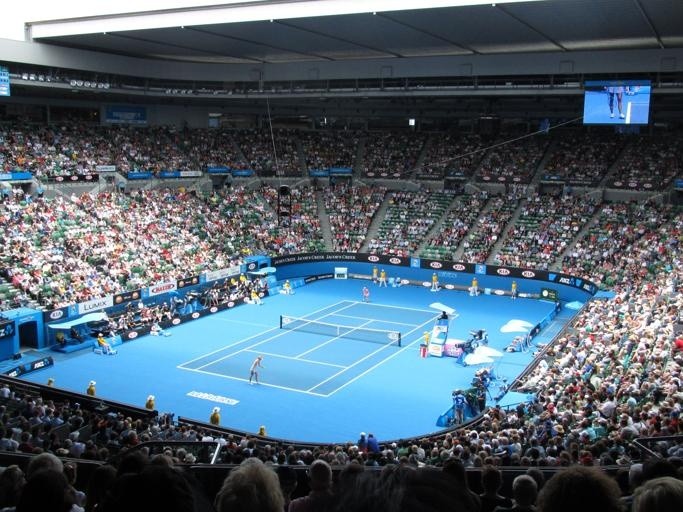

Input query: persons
[0,121,682,511]
[601,86,628,120]
